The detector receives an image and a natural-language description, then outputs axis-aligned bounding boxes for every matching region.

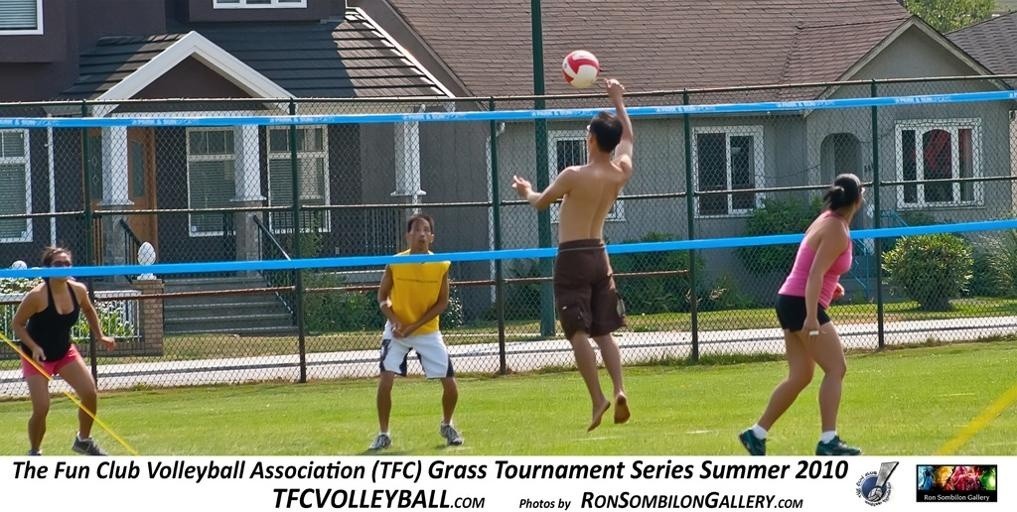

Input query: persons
[12,245,117,456]
[511,78,634,432]
[737,168,864,456]
[367,212,464,451]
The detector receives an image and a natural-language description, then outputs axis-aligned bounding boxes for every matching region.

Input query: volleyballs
[562,49,600,88]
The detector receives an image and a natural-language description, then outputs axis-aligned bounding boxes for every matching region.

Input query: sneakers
[815,433,862,455]
[737,428,768,456]
[367,431,393,451]
[71,431,110,456]
[438,420,465,447]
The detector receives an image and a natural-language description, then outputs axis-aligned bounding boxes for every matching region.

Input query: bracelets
[378,299,393,309]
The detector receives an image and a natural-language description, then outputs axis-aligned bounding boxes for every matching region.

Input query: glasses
[585,124,594,134]
[48,260,71,268]
